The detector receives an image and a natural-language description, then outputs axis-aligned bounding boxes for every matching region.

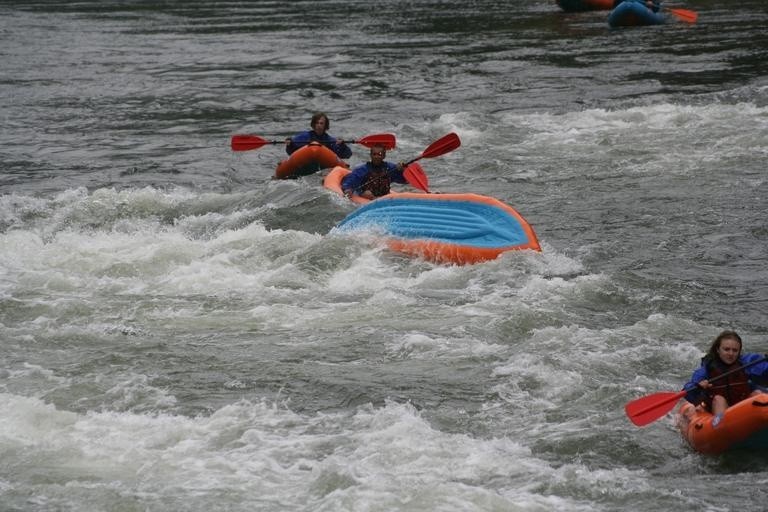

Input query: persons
[684,330,768,417]
[611,0,660,13]
[284,111,353,159]
[340,143,409,200]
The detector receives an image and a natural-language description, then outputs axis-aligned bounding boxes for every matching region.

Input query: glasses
[373,152,382,156]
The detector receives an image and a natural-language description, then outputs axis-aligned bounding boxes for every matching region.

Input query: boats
[331,193,540,268]
[556,0,612,12]
[324,166,398,206]
[676,391,768,456]
[275,140,348,177]
[609,2,665,28]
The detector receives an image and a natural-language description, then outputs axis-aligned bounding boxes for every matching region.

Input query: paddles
[403,163,431,195]
[591,0,699,23]
[231,134,395,151]
[625,355,768,426]
[351,132,460,192]
